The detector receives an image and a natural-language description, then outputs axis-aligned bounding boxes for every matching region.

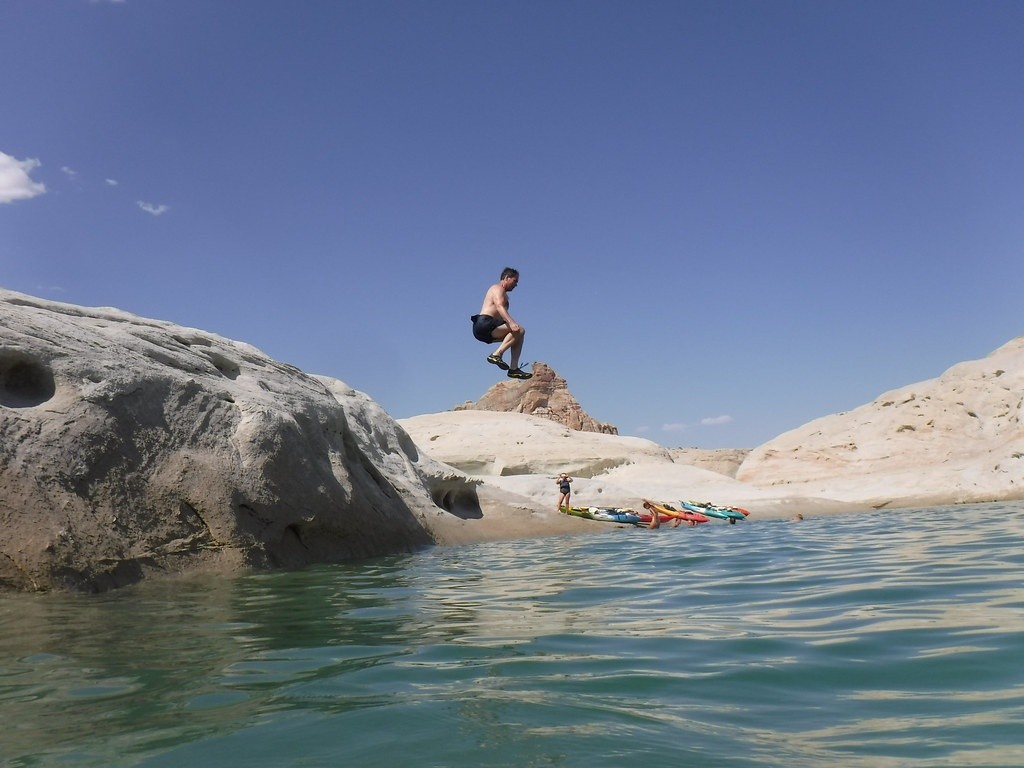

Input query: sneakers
[507,362,532,378]
[487,353,509,370]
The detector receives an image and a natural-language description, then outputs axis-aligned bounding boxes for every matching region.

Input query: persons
[556,473,573,516]
[643,503,661,530]
[472,268,533,379]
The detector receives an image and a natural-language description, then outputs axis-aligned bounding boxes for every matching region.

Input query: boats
[558,505,643,524]
[643,498,711,526]
[678,499,751,524]
[636,513,676,523]
